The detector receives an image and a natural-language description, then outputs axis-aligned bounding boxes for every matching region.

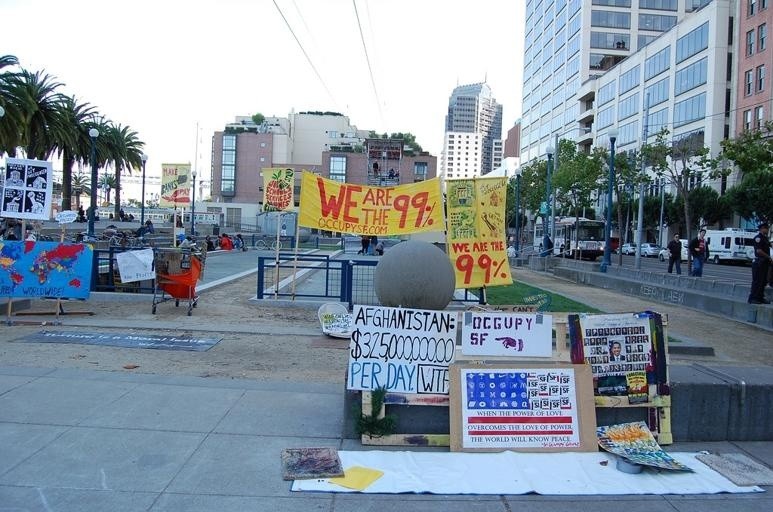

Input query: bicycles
[254,232,282,251]
[46,230,143,247]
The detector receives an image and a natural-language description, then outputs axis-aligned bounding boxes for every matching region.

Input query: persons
[667,234,681,274]
[373,160,399,179]
[689,229,709,277]
[357,236,384,255]
[543,233,553,256]
[0,205,245,252]
[610,342,625,362]
[507,245,516,258]
[748,223,773,303]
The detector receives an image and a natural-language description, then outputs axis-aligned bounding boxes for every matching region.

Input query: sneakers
[750,299,771,304]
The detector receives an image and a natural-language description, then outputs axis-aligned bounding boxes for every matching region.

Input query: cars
[658,239,693,263]
[616,243,636,256]
[634,243,661,258]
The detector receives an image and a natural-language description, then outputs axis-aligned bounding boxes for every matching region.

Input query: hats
[758,223,769,229]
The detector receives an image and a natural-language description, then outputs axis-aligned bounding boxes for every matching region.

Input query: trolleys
[151,247,205,315]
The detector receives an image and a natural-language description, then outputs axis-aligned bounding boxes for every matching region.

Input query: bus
[532,216,606,261]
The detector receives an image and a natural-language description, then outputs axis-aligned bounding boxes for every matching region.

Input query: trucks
[609,237,623,253]
[696,227,760,265]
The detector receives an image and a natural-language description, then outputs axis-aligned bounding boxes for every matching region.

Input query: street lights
[600,126,618,272]
[0,106,4,119]
[514,168,521,258]
[140,154,147,248]
[86,128,98,239]
[543,145,554,257]
[190,171,196,244]
